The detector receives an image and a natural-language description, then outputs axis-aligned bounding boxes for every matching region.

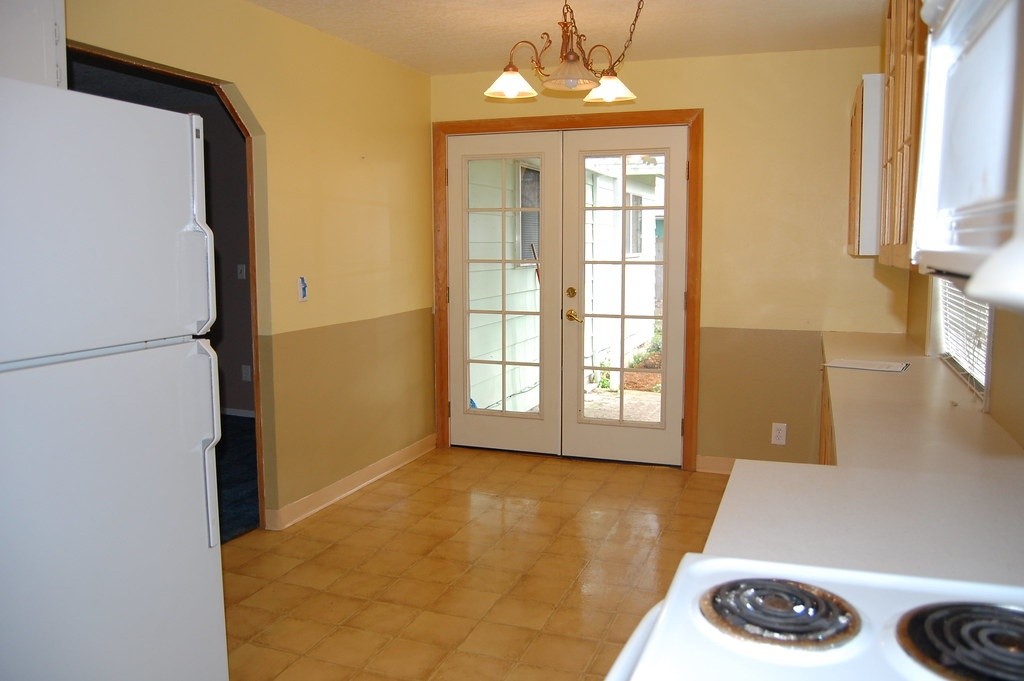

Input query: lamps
[484,0,645,103]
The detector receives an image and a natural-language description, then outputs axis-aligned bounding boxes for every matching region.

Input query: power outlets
[771,422,787,446]
[242,365,251,382]
[237,264,246,280]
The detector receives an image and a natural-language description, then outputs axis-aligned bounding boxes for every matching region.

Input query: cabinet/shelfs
[818,349,837,466]
[847,0,1024,307]
